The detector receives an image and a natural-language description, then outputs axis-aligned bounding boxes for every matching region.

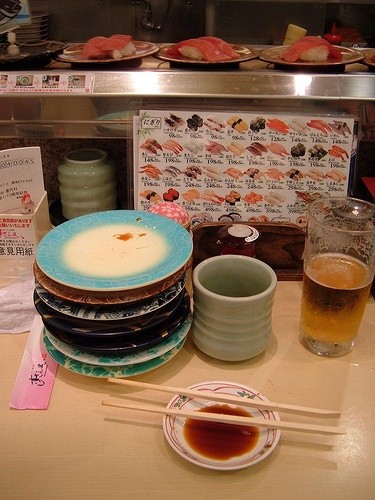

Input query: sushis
[140,113,351,226]
[280,35,342,62]
[167,36,241,61]
[82,34,136,59]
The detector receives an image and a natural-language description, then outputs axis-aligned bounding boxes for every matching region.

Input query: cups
[296,193,375,359]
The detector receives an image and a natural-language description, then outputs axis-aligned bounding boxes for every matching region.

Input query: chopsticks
[100,376,346,436]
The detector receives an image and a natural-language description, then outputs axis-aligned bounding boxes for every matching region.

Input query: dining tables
[0,265,375,500]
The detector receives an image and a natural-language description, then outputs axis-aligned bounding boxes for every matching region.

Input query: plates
[0,39,72,72]
[152,41,261,68]
[32,209,200,382]
[162,380,281,469]
[49,194,127,230]
[54,41,160,66]
[98,109,136,131]
[358,48,375,69]
[9,11,50,45]
[259,44,366,67]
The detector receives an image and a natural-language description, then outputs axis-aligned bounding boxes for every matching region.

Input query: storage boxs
[0,191,50,256]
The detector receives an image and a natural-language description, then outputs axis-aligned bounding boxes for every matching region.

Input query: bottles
[56,148,120,220]
[191,254,279,364]
[212,222,261,262]
